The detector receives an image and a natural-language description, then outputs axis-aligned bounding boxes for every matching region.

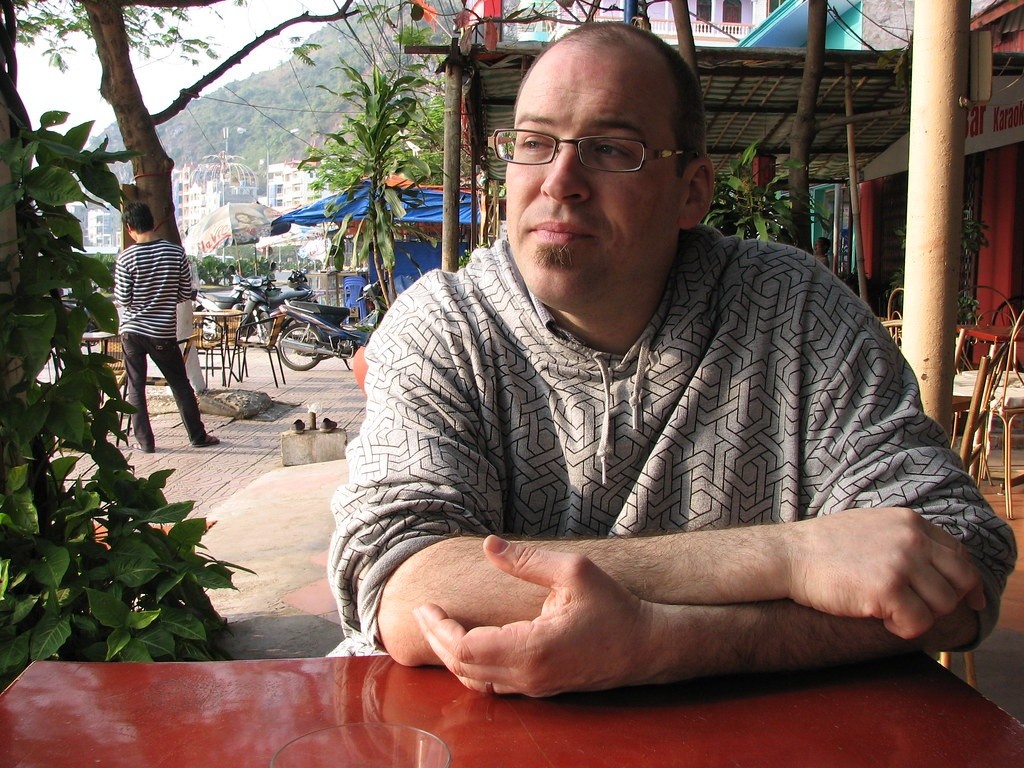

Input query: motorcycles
[278,278,388,373]
[194,261,320,344]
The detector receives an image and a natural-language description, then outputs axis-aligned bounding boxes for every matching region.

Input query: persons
[115,203,221,453]
[322,23,1018,701]
[813,236,832,268]
[231,212,263,227]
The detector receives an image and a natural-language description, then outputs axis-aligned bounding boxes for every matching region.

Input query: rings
[484,682,496,696]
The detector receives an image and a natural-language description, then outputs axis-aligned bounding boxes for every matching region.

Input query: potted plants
[298,52,437,396]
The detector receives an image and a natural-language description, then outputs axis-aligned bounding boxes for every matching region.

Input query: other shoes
[319,418,337,433]
[130,442,155,453]
[290,418,305,434]
[191,435,221,447]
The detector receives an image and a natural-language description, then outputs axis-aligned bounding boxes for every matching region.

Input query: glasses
[487,127,699,172]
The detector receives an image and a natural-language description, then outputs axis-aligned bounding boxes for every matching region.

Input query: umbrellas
[182,201,334,276]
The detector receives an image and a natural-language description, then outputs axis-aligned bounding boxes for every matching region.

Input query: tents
[268,178,490,244]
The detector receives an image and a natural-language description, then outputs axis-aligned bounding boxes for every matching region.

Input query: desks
[956,324,1024,382]
[82,332,116,405]
[0,654,1024,768]
[193,312,248,386]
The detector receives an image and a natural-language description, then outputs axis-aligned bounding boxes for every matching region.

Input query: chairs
[48,309,286,435]
[879,287,1024,519]
